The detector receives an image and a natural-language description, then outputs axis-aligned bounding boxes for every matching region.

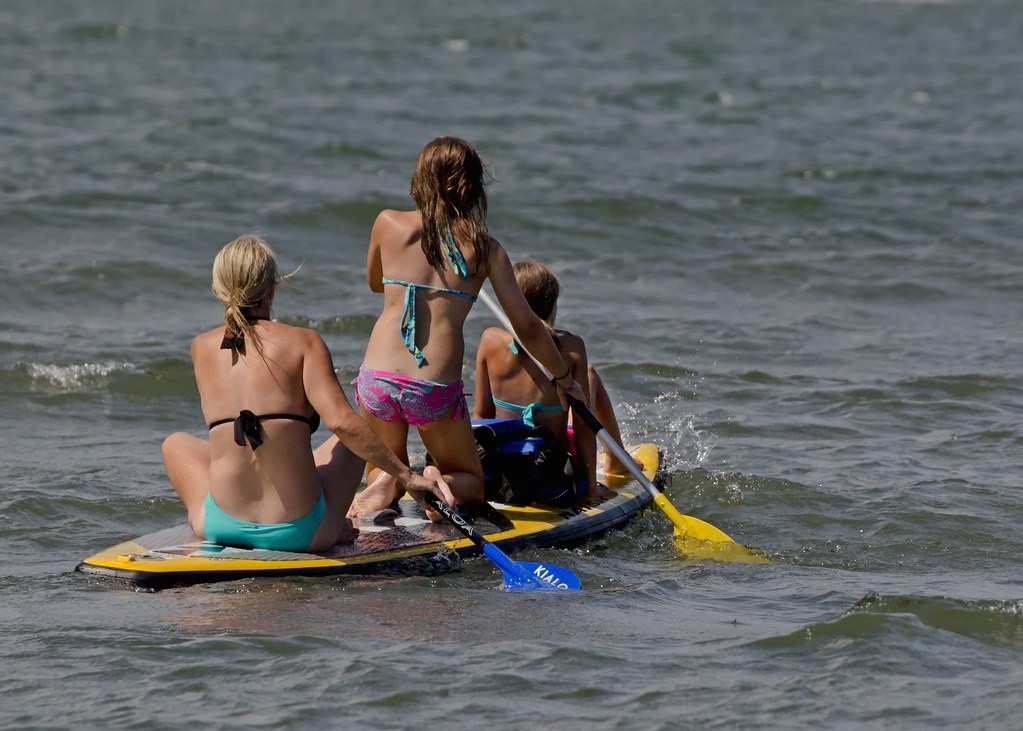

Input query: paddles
[477,286,771,565]
[421,488,583,592]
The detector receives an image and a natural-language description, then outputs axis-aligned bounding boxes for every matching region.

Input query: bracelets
[555,367,570,380]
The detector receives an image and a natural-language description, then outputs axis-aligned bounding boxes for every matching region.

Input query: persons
[161,236,447,553]
[475,259,641,502]
[347,137,587,522]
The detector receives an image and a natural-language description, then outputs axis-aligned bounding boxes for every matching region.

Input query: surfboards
[79,442,666,586]
